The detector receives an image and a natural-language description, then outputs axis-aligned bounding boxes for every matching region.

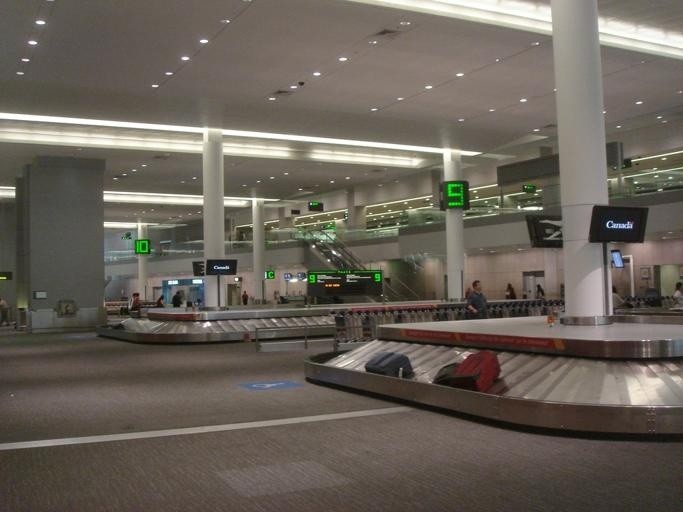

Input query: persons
[242,290,248,306]
[127,292,138,312]
[612,285,625,310]
[535,283,544,297]
[0,296,11,326]
[465,287,471,297]
[466,278,491,319]
[156,294,165,307]
[171,291,183,307]
[194,298,201,305]
[133,294,142,308]
[673,282,683,300]
[504,282,514,299]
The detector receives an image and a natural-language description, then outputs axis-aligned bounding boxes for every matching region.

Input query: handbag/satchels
[365,351,412,379]
[433,349,502,394]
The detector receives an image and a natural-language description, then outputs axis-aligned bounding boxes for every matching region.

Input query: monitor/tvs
[588,205,649,244]
[206,259,237,275]
[525,214,563,248]
[192,261,204,276]
[611,249,624,268]
